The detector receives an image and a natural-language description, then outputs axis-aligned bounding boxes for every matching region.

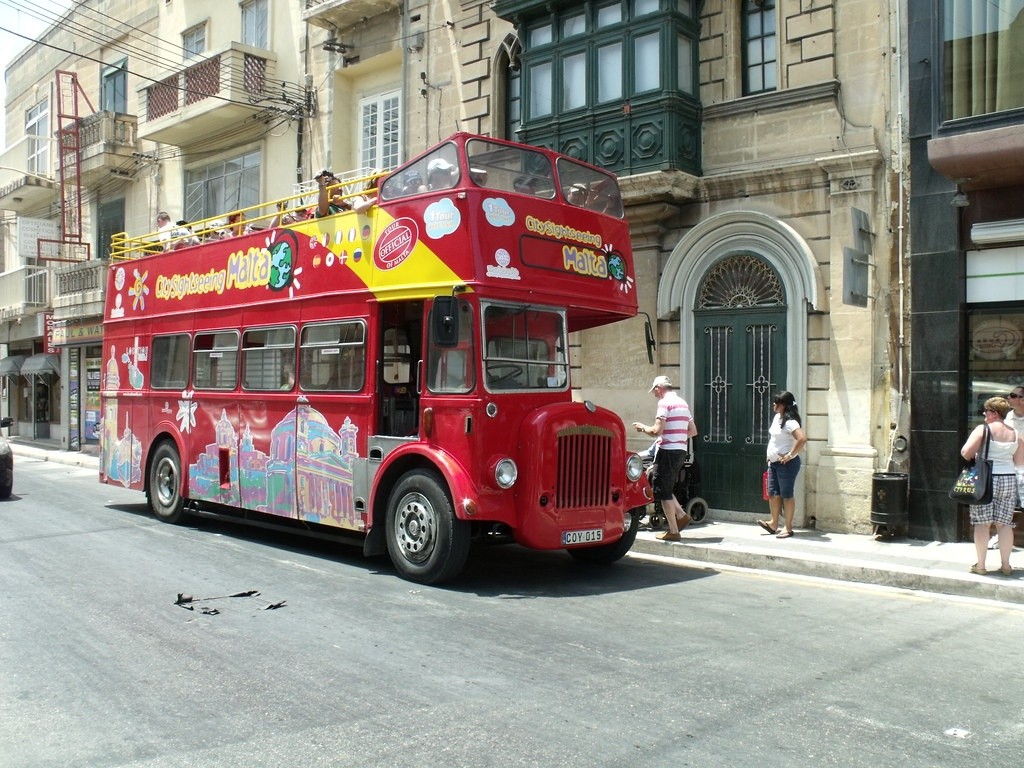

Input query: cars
[0,415,14,500]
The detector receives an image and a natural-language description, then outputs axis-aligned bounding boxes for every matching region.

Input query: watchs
[642,425,646,431]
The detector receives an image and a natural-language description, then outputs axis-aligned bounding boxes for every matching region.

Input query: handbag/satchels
[762,472,770,500]
[949,425,993,505]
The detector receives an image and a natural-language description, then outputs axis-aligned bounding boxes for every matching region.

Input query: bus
[96,132,656,586]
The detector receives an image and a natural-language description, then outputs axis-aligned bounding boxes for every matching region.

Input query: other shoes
[988,534,1000,549]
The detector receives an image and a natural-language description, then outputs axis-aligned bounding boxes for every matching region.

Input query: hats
[648,376,671,394]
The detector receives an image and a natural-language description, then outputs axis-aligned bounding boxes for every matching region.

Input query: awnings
[19,352,62,386]
[0,354,32,387]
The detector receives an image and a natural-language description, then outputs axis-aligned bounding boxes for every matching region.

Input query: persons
[268,200,308,230]
[960,394,1024,575]
[350,158,487,214]
[218,212,252,240]
[987,385,1024,550]
[157,211,201,254]
[567,182,617,215]
[280,364,316,390]
[757,389,807,538]
[436,329,492,391]
[314,169,352,218]
[632,375,697,540]
[512,175,538,196]
[205,218,231,245]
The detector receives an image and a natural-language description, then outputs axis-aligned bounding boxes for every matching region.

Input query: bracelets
[788,452,793,459]
[319,183,326,188]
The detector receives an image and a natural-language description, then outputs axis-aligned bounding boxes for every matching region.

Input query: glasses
[1010,392,1024,398]
[985,409,995,413]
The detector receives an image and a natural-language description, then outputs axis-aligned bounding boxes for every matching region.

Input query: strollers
[637,453,708,531]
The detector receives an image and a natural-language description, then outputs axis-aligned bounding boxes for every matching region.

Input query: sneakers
[677,514,693,532]
[655,530,681,541]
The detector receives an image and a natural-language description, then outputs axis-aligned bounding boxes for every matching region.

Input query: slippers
[1000,565,1012,575]
[757,520,775,534]
[971,563,987,574]
[776,531,794,538]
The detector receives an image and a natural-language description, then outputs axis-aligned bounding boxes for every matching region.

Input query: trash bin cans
[871,471,909,526]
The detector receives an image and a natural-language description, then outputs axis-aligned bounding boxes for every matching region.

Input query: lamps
[950,183,970,207]
